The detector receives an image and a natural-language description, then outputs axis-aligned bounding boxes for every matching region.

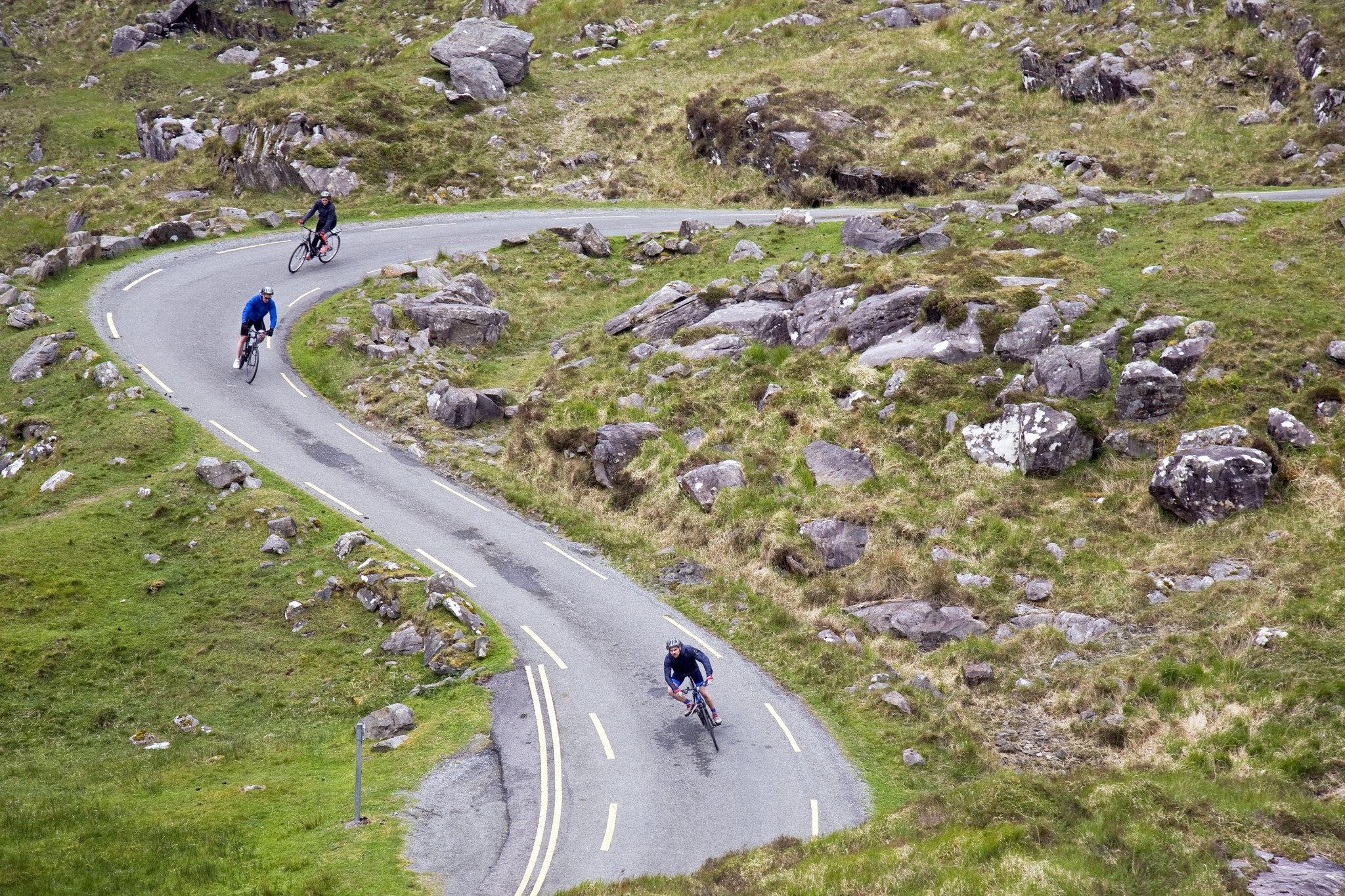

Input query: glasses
[264,295,272,299]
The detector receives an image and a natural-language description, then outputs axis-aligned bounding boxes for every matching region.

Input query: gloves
[241,323,248,332]
[298,220,304,225]
[707,676,714,685]
[320,231,325,236]
[266,328,274,337]
[673,688,683,697]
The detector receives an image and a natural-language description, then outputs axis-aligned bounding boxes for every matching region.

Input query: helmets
[666,637,681,650]
[261,286,274,295]
[320,191,331,200]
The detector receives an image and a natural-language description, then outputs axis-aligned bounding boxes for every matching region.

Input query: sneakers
[302,253,311,259]
[684,700,697,717]
[319,243,330,253]
[712,712,722,725]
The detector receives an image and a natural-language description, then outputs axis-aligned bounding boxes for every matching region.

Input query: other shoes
[253,350,257,355]
[233,359,240,368]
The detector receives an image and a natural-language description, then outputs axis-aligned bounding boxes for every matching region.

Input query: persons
[664,637,722,725]
[233,286,277,369]
[298,191,337,259]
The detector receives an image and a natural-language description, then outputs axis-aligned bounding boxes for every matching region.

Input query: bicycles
[673,679,719,752]
[288,224,342,273]
[242,327,270,384]
